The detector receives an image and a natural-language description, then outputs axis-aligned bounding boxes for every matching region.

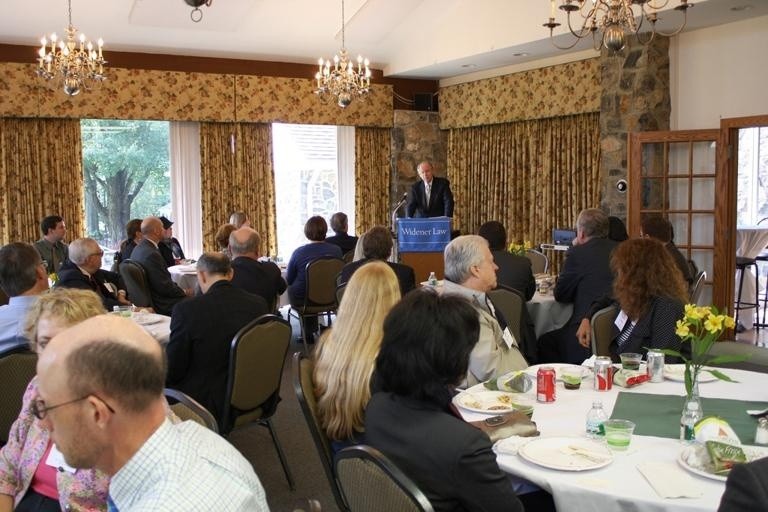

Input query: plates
[518,437,612,473]
[456,391,512,414]
[526,363,592,379]
[134,313,162,325]
[667,365,719,381]
[678,451,728,481]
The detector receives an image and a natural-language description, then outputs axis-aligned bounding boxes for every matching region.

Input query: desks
[735,227,768,328]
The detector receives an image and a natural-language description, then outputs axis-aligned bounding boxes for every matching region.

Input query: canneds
[594,356,613,392]
[537,367,557,403]
[646,349,665,383]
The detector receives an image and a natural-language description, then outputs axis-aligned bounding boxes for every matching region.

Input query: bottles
[539,280,548,295]
[428,272,438,286]
[587,403,608,438]
[680,402,702,445]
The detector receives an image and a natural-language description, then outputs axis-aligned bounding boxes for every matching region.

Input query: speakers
[413,91,432,111]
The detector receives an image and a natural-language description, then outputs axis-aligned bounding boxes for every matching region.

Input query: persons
[420,235,528,390]
[719,457,768,512]
[576,238,693,364]
[480,221,536,302]
[1,242,49,351]
[30,313,273,512]
[35,211,414,343]
[313,259,404,442]
[2,286,115,511]
[364,288,525,510]
[164,251,270,423]
[609,216,689,282]
[538,208,620,364]
[403,157,456,219]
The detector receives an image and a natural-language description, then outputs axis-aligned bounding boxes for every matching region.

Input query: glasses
[38,259,49,270]
[28,393,117,420]
[84,249,103,263]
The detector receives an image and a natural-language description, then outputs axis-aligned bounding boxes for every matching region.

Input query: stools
[735,253,768,335]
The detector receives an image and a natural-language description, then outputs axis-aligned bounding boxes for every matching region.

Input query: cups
[512,396,536,419]
[619,353,643,371]
[561,365,583,390]
[606,419,635,447]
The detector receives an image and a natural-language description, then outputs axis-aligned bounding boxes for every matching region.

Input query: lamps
[541,1,699,58]
[35,3,109,97]
[312,2,373,110]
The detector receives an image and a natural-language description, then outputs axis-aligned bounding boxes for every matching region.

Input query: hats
[158,216,174,230]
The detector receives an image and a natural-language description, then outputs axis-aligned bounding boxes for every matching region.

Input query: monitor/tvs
[552,229,576,243]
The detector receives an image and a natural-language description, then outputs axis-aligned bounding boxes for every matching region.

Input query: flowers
[656,303,753,399]
[506,237,531,257]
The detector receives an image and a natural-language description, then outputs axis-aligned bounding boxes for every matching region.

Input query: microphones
[397,192,408,205]
